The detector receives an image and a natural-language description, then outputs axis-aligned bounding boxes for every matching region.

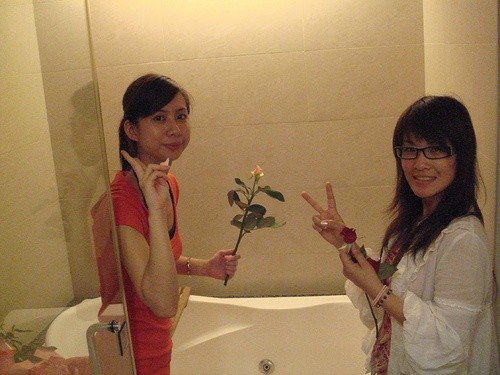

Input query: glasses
[393,144,457,159]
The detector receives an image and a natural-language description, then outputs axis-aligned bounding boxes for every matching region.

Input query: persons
[59,82,108,297]
[91,73,242,375]
[300,95,500,374]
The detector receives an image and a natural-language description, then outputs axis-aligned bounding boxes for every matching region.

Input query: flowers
[338,227,398,338]
[224,165,286,286]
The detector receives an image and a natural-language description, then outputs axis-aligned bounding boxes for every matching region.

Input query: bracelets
[186,257,191,276]
[374,285,392,308]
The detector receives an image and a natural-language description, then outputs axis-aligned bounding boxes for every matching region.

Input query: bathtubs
[45,294,366,375]
